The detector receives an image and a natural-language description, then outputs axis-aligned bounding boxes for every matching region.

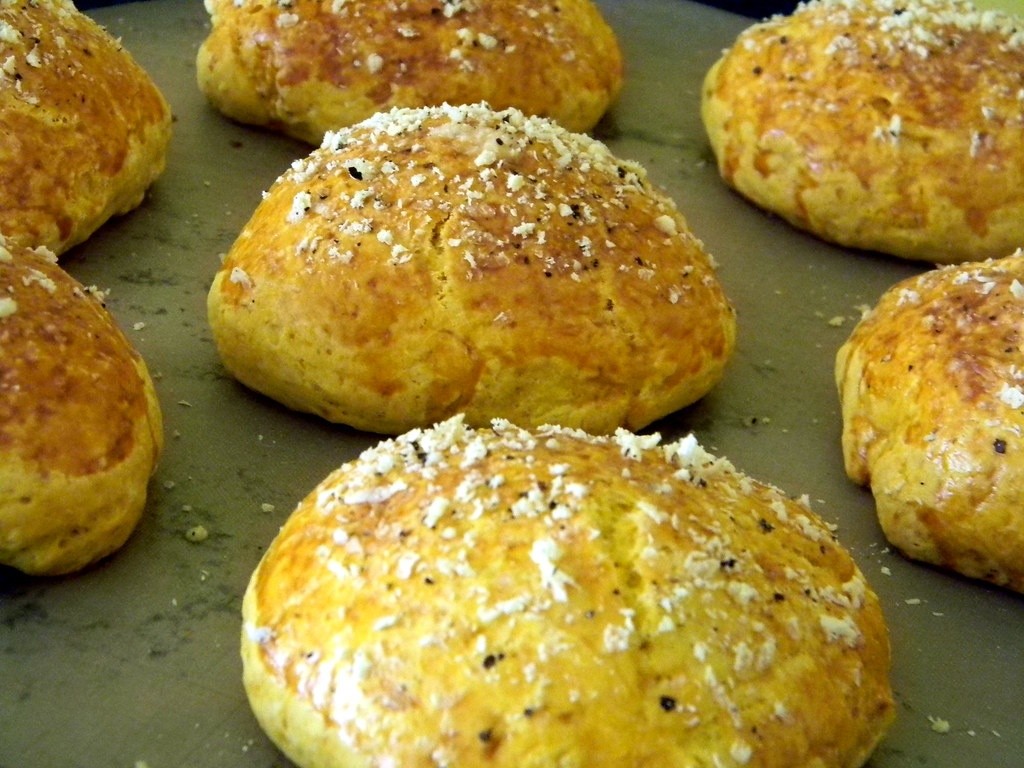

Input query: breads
[0,0,1024,768]
